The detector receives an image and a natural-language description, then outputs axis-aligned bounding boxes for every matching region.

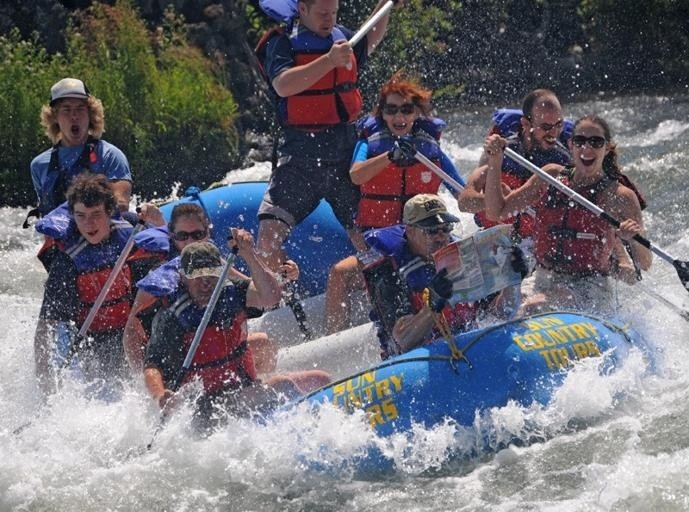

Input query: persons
[247,1,404,310]
[362,192,530,361]
[122,203,301,375]
[30,78,134,243]
[34,171,168,411]
[457,90,649,240]
[481,114,653,321]
[143,227,333,441]
[325,72,468,337]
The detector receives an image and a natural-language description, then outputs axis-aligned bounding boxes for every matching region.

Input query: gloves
[422,267,453,311]
[509,250,529,278]
[388,138,416,163]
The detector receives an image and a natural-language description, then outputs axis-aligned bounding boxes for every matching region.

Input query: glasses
[570,134,605,149]
[379,102,415,114]
[536,119,565,129]
[167,229,209,240]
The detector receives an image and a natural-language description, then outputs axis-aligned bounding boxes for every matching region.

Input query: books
[431,223,522,311]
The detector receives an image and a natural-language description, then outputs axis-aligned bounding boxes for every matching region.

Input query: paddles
[502,146,689,290]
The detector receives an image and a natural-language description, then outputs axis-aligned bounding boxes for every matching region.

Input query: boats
[33,180,656,482]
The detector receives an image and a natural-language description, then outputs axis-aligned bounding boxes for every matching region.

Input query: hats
[404,194,460,228]
[48,78,90,104]
[178,241,229,281]
